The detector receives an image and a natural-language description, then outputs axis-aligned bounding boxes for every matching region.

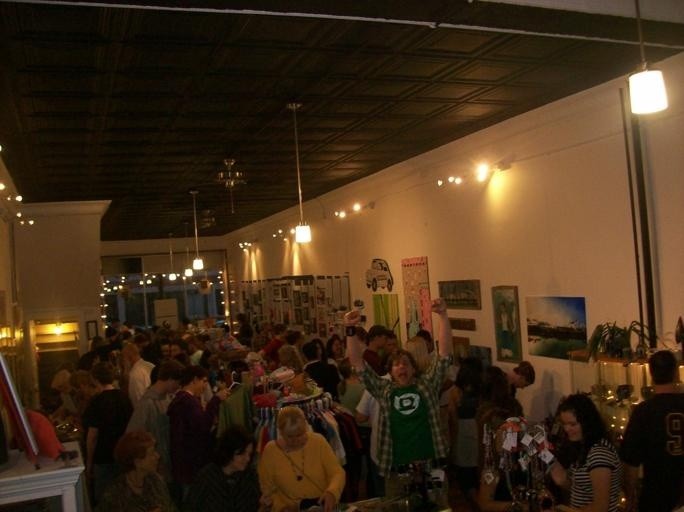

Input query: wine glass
[396,462,416,507]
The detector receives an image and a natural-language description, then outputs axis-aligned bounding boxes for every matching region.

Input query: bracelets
[343,325,356,337]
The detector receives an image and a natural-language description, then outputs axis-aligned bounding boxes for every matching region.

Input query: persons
[179,424,256,511]
[96,427,175,511]
[341,298,456,511]
[49,313,536,499]
[548,392,619,511]
[619,349,682,511]
[259,406,349,511]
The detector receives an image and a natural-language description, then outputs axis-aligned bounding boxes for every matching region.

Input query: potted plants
[584,316,662,365]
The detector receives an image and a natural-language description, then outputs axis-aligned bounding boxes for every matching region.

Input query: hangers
[252,392,342,430]
[225,371,244,393]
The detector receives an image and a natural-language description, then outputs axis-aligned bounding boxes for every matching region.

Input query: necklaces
[284,446,305,481]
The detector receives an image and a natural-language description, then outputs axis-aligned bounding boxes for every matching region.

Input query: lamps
[627,0,668,115]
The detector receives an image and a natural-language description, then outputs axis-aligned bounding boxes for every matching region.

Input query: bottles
[478,423,501,486]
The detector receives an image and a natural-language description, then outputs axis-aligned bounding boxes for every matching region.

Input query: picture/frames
[491,284,523,365]
[436,279,482,311]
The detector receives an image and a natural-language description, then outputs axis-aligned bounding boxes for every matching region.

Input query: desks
[0,440,85,511]
